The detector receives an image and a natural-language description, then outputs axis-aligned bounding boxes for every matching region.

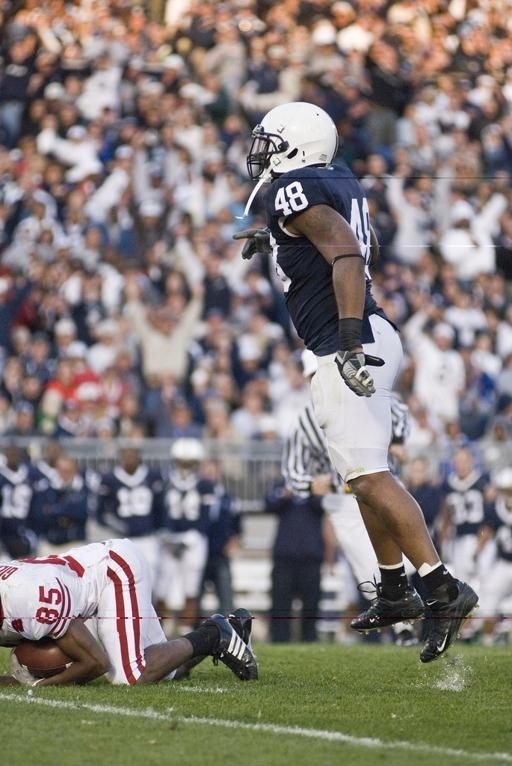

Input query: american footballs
[13,638,73,678]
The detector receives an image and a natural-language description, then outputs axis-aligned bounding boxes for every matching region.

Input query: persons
[232,102,479,664]
[0,535,260,685]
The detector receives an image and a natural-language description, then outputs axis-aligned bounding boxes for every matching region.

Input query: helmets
[171,438,205,461]
[246,102,338,183]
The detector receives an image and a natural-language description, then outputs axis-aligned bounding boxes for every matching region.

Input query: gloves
[233,226,273,260]
[334,350,385,397]
[11,654,44,686]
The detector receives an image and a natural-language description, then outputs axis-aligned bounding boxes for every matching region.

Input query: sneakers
[202,608,258,680]
[350,581,479,663]
